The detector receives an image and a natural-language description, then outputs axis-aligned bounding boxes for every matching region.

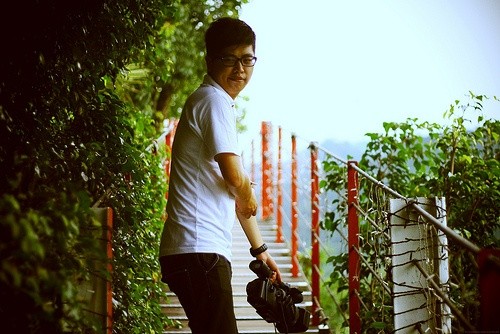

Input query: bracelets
[250,243,268,257]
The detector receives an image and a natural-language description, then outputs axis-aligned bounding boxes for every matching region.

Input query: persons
[158,17,283,334]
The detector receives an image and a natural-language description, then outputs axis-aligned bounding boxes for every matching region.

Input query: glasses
[210,55,257,67]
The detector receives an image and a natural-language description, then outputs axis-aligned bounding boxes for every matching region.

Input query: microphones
[282,282,303,304]
